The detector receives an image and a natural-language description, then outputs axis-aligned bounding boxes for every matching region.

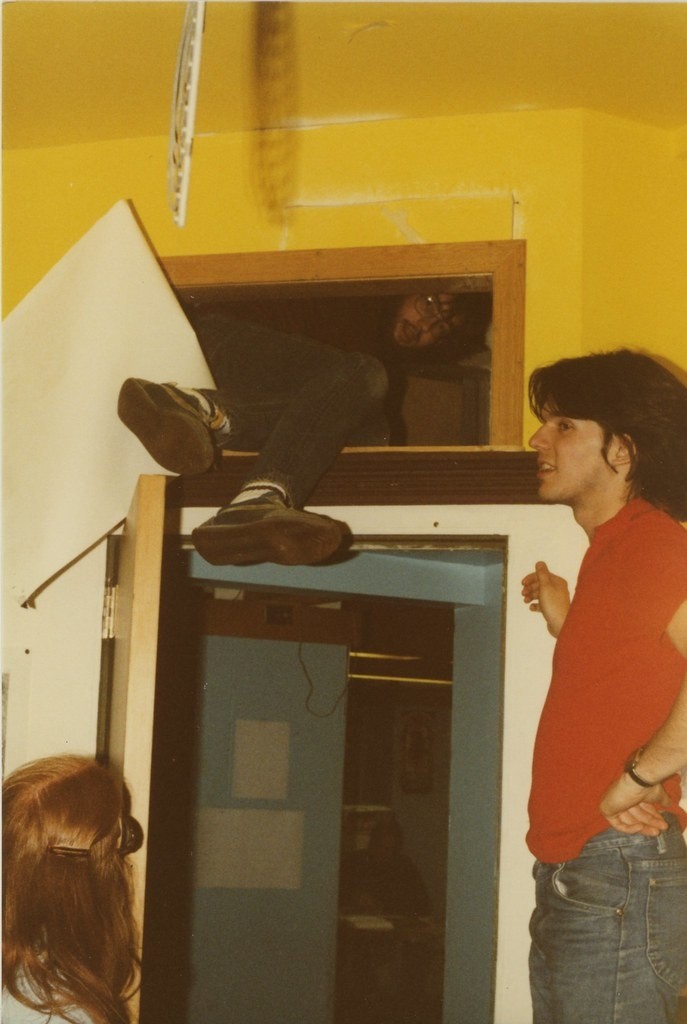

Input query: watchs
[624,748,657,788]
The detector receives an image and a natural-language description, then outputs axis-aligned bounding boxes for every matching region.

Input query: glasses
[414,292,454,345]
[48,816,144,863]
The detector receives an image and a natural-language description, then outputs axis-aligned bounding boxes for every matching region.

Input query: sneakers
[117,378,223,475]
[191,491,352,566]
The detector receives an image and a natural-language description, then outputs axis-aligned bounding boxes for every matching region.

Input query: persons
[2,754,144,1024]
[116,293,475,566]
[522,348,687,1023]
